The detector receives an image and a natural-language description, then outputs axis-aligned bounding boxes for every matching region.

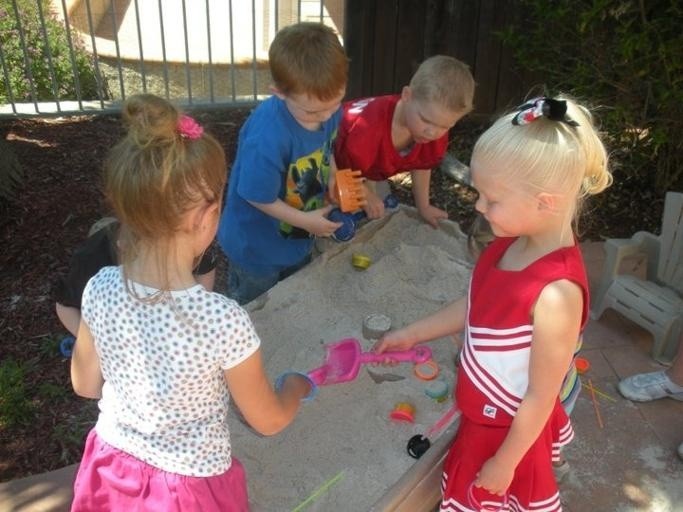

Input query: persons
[552,333,583,484]
[216,22,350,307]
[69,94,318,512]
[52,218,219,340]
[616,336,683,460]
[370,95,612,512]
[333,55,475,229]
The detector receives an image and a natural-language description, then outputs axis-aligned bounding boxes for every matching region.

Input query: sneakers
[616,371,682,402]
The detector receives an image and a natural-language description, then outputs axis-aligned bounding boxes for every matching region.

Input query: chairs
[590,191,683,366]
